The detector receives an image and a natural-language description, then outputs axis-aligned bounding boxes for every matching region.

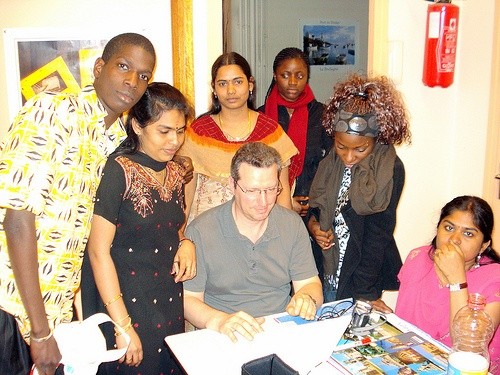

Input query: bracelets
[179,239,195,245]
[103,293,123,307]
[29,328,53,343]
[114,317,132,337]
[303,294,316,305]
[112,315,129,325]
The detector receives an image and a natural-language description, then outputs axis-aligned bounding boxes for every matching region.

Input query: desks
[164,297,492,375]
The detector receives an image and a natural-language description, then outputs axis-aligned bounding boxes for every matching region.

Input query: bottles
[447,293,494,375]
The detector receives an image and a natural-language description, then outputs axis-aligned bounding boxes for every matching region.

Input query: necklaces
[288,112,293,118]
[218,109,252,141]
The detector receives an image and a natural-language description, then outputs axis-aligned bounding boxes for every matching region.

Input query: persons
[0,33,500,375]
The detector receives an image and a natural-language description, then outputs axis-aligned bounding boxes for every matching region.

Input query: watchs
[446,283,468,291]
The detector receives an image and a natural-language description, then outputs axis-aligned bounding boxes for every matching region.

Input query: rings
[322,242,325,246]
[173,261,178,264]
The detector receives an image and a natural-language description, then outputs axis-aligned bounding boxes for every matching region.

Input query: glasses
[235,179,283,197]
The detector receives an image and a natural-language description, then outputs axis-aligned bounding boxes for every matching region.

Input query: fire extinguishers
[422,0,461,88]
